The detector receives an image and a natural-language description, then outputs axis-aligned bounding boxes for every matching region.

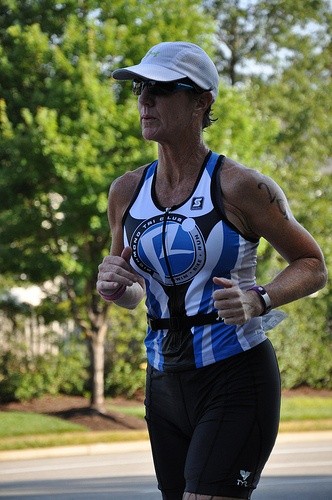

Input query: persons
[96,41,328,500]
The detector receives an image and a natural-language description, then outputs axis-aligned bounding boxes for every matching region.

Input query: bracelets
[99,284,126,301]
[247,286,273,316]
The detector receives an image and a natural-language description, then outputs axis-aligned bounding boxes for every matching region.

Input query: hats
[115,43,222,97]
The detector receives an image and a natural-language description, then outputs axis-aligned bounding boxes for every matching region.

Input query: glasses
[130,79,194,97]
